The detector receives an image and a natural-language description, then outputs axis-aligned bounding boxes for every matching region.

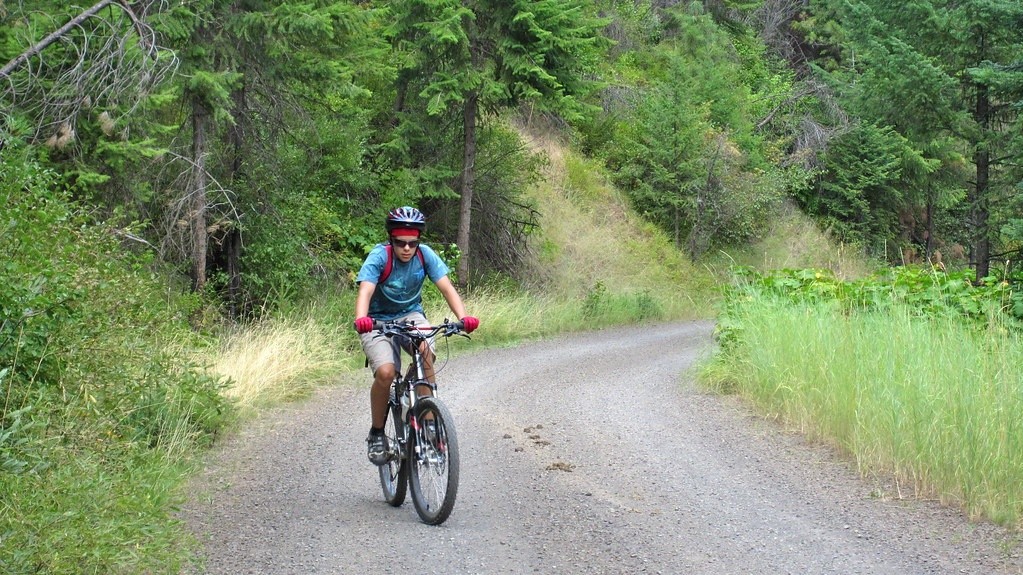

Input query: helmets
[386,206,426,235]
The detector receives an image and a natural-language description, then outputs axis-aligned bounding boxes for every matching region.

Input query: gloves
[461,316,479,334]
[354,317,373,333]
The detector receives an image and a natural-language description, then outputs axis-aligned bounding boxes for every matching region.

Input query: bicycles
[352,318,473,527]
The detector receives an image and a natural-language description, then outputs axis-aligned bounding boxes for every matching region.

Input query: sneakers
[422,419,436,444]
[367,431,386,466]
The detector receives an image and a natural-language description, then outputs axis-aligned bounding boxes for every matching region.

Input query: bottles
[401,391,410,423]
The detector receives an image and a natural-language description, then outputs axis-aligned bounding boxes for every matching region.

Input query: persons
[354,206,480,465]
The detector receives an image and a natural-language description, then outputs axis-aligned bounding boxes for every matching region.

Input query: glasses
[392,237,419,247]
